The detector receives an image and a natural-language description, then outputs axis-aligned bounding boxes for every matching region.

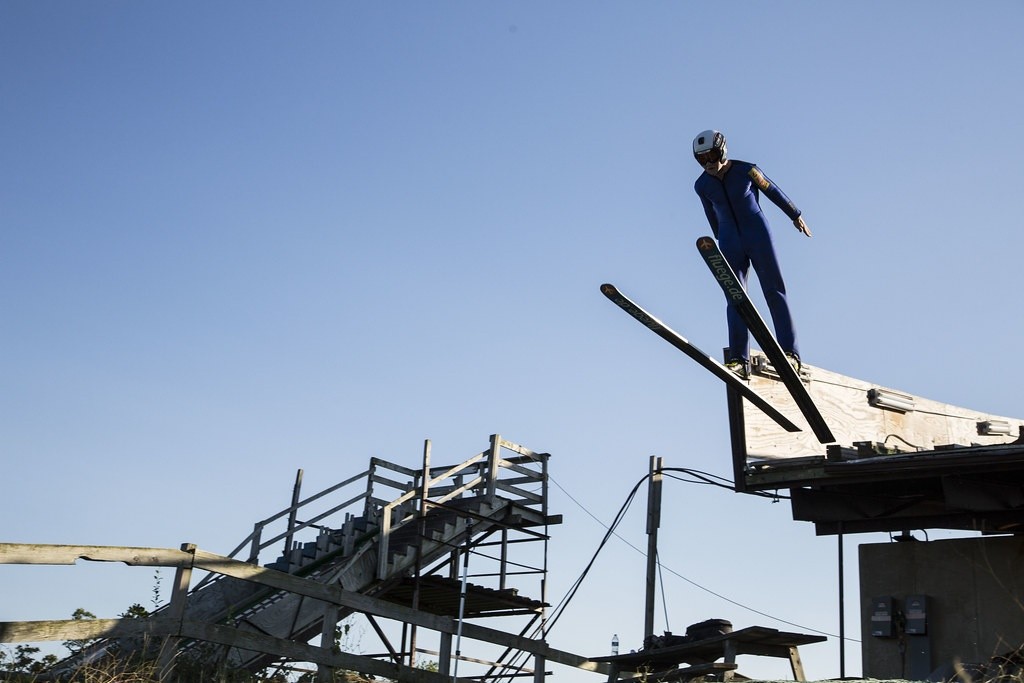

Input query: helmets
[693,129,727,164]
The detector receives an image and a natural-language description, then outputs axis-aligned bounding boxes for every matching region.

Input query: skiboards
[600,283,802,435]
[695,235,836,446]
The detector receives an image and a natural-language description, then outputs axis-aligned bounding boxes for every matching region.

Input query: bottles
[611,633,619,656]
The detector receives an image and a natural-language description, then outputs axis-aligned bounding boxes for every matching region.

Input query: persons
[693,130,812,380]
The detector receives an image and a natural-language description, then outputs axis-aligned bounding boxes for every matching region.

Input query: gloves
[794,216,811,236]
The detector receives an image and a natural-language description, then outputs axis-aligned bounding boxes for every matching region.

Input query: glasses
[698,148,720,165]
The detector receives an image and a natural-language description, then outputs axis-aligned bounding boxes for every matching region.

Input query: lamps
[867,389,915,412]
[980,421,1010,435]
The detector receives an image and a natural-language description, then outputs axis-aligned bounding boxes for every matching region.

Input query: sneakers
[785,351,802,376]
[723,361,753,381]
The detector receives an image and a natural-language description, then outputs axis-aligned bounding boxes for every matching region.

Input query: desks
[588,626,827,683]
